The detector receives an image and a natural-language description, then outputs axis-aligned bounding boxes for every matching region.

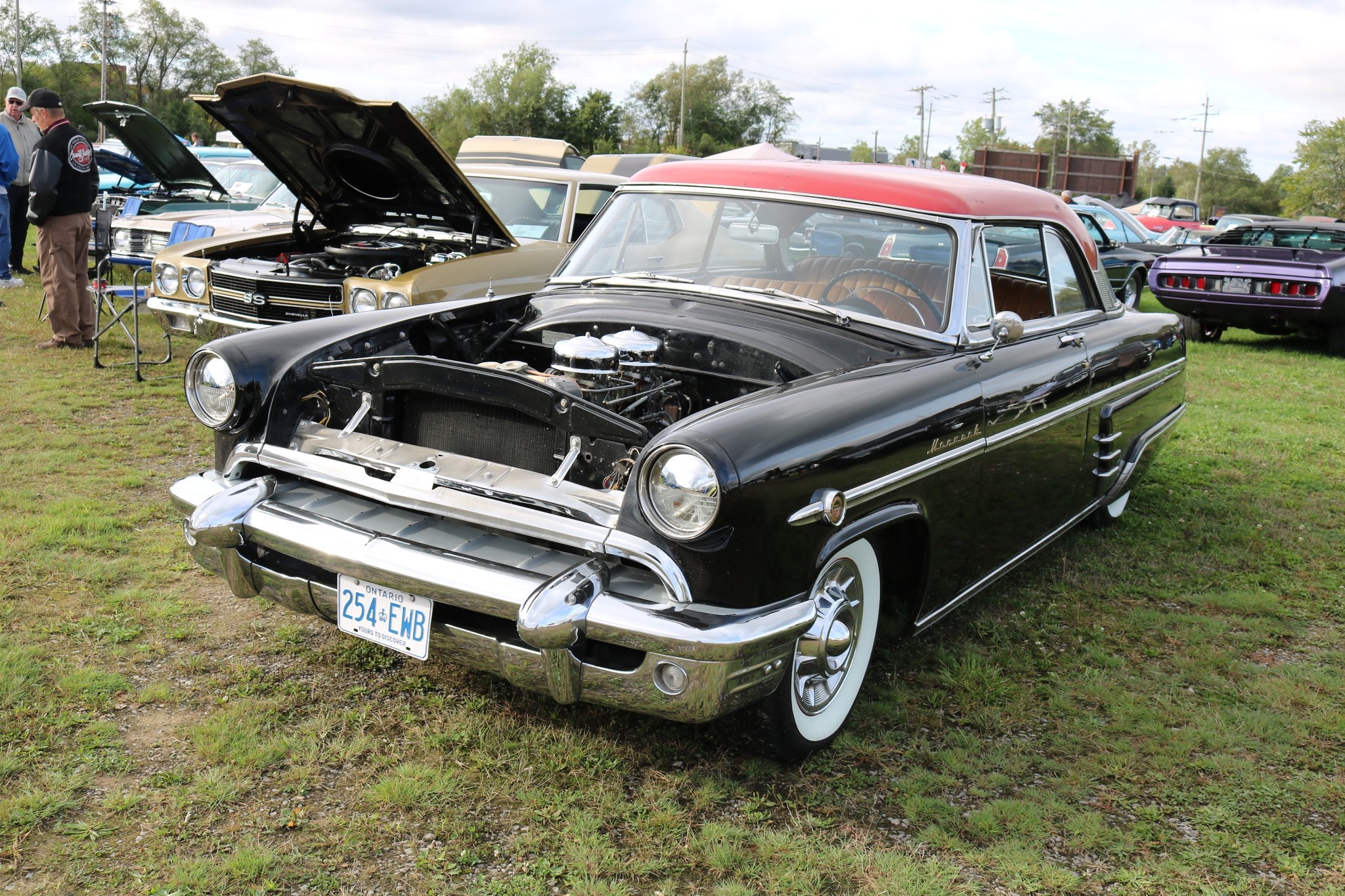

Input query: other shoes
[35,337,94,350]
[8,264,36,275]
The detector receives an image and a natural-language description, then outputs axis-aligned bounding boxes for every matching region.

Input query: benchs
[781,245,1080,340]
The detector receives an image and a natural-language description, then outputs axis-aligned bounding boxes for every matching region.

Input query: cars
[166,161,1192,756]
[81,72,1345,353]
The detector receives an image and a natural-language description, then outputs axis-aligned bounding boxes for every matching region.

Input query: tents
[216,130,242,144]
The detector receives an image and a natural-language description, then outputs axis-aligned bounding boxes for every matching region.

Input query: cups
[92,278,108,297]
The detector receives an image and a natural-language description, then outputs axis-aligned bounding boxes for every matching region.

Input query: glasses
[7,98,23,105]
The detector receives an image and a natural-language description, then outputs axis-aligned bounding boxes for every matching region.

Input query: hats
[17,88,62,112]
[6,87,26,103]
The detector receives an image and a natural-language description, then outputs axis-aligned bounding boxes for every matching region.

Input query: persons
[1060,189,1078,205]
[0,87,43,274]
[17,87,100,350]
[190,132,203,147]
[0,124,25,287]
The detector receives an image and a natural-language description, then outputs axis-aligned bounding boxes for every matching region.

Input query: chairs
[852,289,992,343]
[708,269,853,318]
[489,194,604,253]
[82,193,142,248]
[37,210,115,320]
[1255,232,1336,251]
[84,198,145,237]
[89,222,217,385]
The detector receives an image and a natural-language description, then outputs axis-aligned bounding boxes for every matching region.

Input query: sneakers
[0,276,24,288]
